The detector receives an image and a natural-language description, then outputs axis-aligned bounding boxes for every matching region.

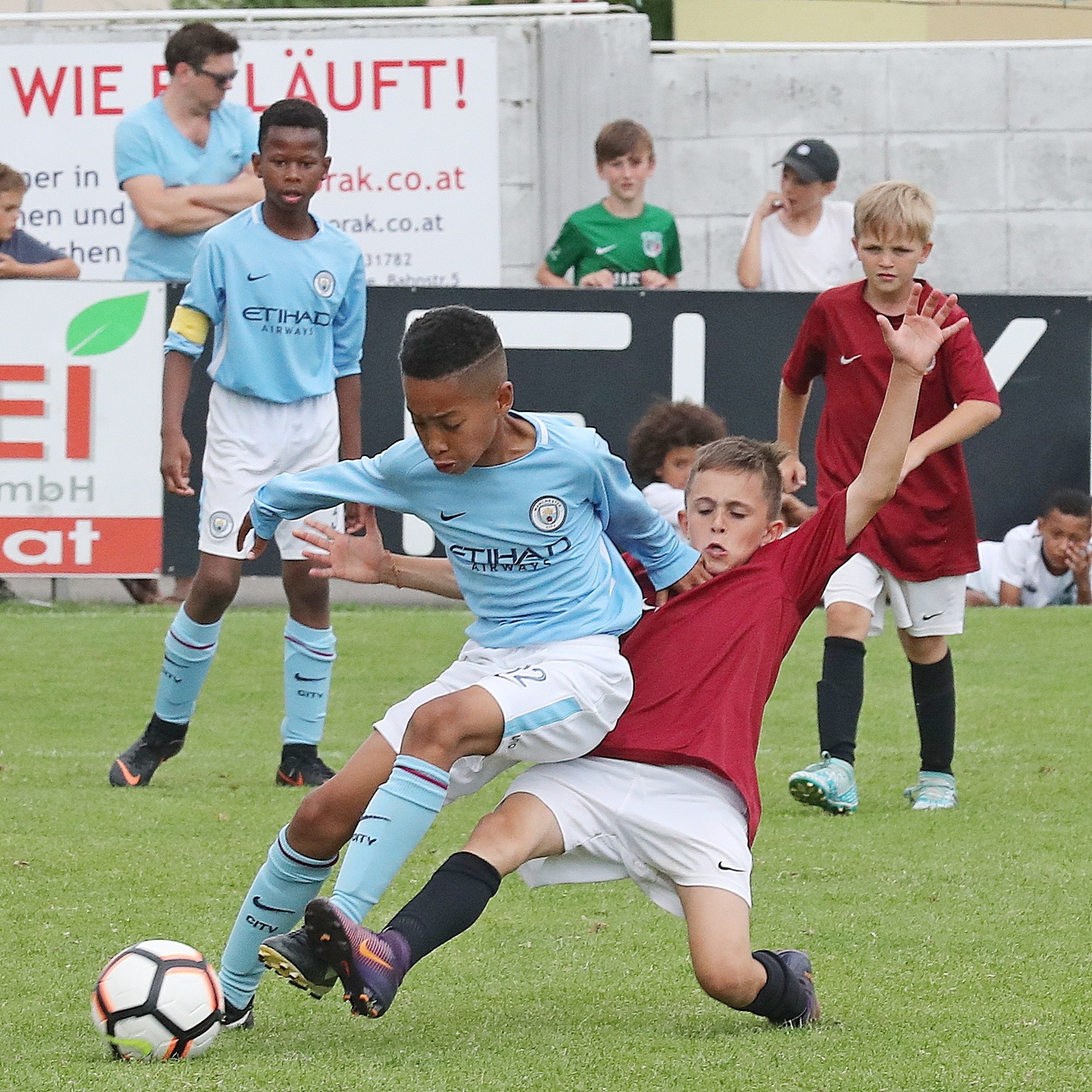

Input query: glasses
[192,64,237,84]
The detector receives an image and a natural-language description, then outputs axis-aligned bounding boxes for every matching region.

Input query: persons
[536,118,682,291]
[108,98,368,789]
[291,283,969,1028]
[217,305,712,1029]
[0,162,81,600]
[112,24,266,604]
[777,181,1002,816]
[736,139,867,292]
[965,488,1092,607]
[625,391,727,545]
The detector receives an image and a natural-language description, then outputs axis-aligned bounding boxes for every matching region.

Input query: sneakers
[786,750,858,815]
[109,725,186,787]
[222,998,254,1028]
[778,946,821,1024]
[258,925,339,1000]
[905,776,955,809]
[274,758,336,786]
[304,896,404,1020]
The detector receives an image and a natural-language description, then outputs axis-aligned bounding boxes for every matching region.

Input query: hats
[772,139,838,183]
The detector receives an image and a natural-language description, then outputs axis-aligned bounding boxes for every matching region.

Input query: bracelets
[385,548,403,588]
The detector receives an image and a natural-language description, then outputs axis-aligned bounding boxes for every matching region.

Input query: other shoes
[125,577,162,603]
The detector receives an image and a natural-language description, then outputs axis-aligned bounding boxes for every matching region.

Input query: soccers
[89,938,225,1063]
